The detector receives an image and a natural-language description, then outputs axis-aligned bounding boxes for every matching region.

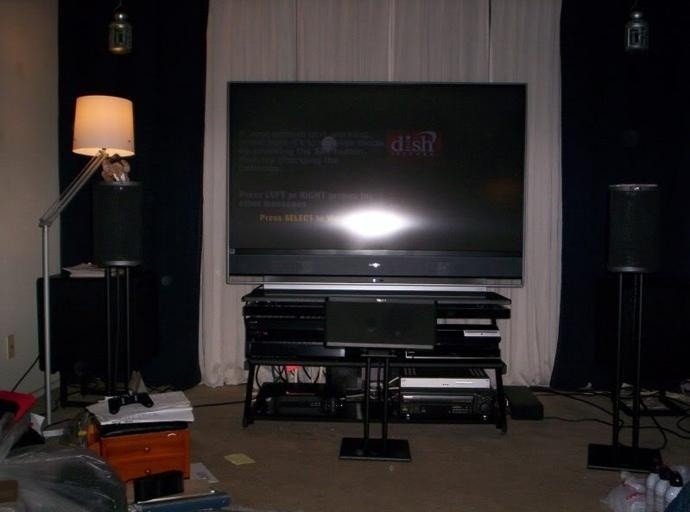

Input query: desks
[126,481,231,512]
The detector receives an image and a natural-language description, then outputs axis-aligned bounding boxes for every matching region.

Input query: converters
[504,387,543,420]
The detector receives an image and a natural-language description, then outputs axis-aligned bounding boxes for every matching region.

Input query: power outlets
[8,335,15,360]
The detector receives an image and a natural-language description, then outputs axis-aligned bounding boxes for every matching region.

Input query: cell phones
[126,470,185,505]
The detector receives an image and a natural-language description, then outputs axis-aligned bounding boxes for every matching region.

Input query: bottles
[623,13,652,50]
[107,13,133,52]
[645,464,683,511]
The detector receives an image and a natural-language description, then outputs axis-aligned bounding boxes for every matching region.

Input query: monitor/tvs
[225,80,526,294]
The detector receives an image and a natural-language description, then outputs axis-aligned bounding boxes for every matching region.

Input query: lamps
[38,95,136,437]
[623,0,650,54]
[106,1,134,56]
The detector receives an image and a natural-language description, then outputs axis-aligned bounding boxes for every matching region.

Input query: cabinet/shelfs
[84,421,191,503]
[240,284,511,436]
[37,264,143,408]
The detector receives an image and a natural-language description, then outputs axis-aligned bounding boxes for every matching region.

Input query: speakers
[93,180,143,268]
[325,294,437,351]
[609,184,660,274]
